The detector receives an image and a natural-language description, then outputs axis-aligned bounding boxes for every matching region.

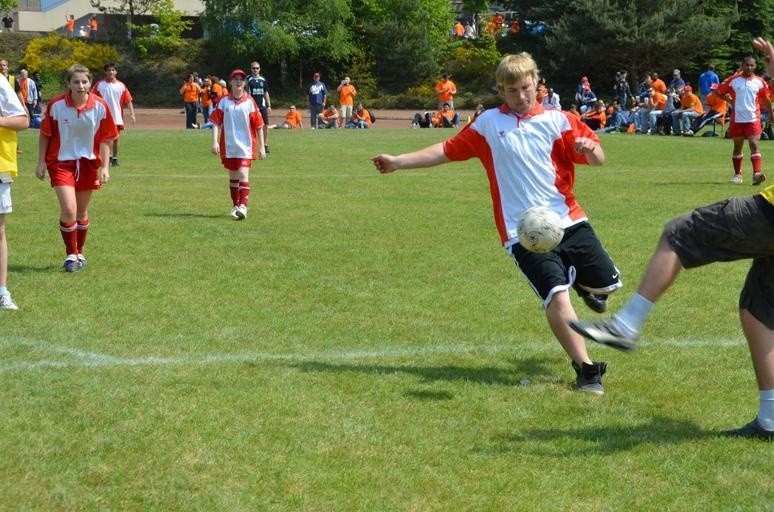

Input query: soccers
[517,204,565,253]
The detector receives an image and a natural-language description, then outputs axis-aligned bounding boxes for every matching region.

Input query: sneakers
[752,172,766,185]
[572,283,608,313]
[110,154,119,166]
[231,204,248,219]
[63,253,86,272]
[572,360,607,395]
[731,174,742,184]
[647,128,695,136]
[568,320,637,354]
[192,120,202,129]
[720,415,774,442]
[0,290,19,310]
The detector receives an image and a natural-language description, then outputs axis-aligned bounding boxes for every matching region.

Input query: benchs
[689,117,731,137]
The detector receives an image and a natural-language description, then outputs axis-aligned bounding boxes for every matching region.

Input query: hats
[230,70,246,79]
[709,83,718,90]
[684,85,692,91]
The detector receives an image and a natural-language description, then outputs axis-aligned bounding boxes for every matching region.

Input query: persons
[36,63,119,272]
[180,72,229,130]
[410,73,460,128]
[245,62,272,154]
[268,105,303,130]
[374,51,623,395]
[718,53,771,187]
[539,64,773,140]
[2,13,15,32]
[567,38,774,440]
[210,70,266,220]
[66,15,74,38]
[90,62,135,166]
[1,60,42,312]
[474,103,485,119]
[89,15,98,40]
[305,73,372,130]
[454,11,520,40]
